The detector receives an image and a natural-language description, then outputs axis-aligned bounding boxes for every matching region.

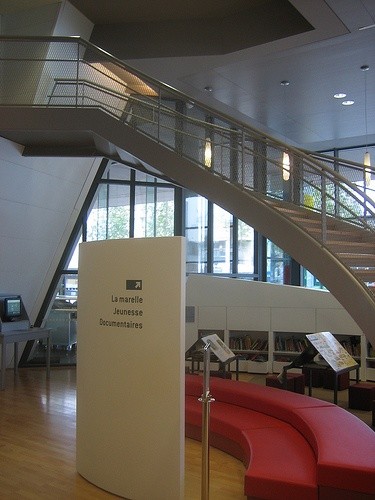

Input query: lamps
[203,86,213,168]
[360,65,371,187]
[279,80,291,181]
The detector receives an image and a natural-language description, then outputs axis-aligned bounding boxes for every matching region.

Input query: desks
[0,328,54,392]
[184,351,239,380]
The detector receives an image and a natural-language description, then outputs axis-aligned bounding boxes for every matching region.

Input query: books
[187,329,374,368]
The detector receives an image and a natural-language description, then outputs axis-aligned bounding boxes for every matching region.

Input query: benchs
[184,373,375,500]
[185,393,319,500]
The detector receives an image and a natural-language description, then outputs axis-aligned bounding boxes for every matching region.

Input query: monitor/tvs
[5,297,22,317]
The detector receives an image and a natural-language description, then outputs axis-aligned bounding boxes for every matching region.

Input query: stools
[301,360,350,391]
[266,373,295,393]
[286,373,305,396]
[348,383,375,413]
